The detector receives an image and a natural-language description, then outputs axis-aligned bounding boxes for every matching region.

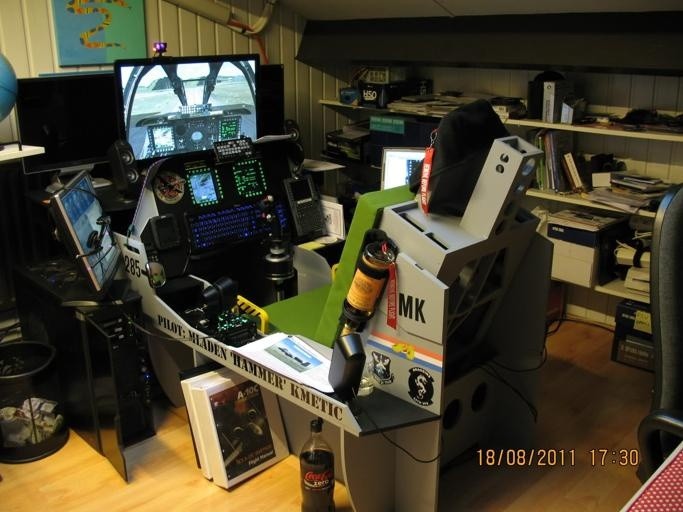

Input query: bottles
[332,244,395,338]
[299,419,337,511]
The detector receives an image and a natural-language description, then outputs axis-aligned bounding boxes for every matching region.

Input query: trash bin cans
[1,342,69,464]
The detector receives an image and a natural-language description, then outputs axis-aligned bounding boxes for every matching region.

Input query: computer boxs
[81,314,158,449]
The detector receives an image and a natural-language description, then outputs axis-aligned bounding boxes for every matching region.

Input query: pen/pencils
[430,101,463,106]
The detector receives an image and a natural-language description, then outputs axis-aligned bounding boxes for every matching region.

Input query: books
[526,127,674,213]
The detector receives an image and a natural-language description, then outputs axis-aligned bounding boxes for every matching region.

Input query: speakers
[330,333,366,415]
[138,213,181,249]
[258,63,288,168]
[108,136,141,195]
[283,118,305,166]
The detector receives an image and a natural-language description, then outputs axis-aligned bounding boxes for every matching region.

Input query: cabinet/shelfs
[318,95,683,327]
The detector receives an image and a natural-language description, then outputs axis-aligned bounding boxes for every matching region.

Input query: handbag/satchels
[409,100,510,216]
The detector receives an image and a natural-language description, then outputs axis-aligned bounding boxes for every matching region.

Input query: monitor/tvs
[13,72,125,195]
[113,53,264,165]
[379,146,427,190]
[49,169,132,308]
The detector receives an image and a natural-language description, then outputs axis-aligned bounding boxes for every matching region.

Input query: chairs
[636,181,681,489]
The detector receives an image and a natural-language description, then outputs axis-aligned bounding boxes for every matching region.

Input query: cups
[234,424,263,453]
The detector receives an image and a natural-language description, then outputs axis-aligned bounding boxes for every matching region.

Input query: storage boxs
[610,298,657,374]
